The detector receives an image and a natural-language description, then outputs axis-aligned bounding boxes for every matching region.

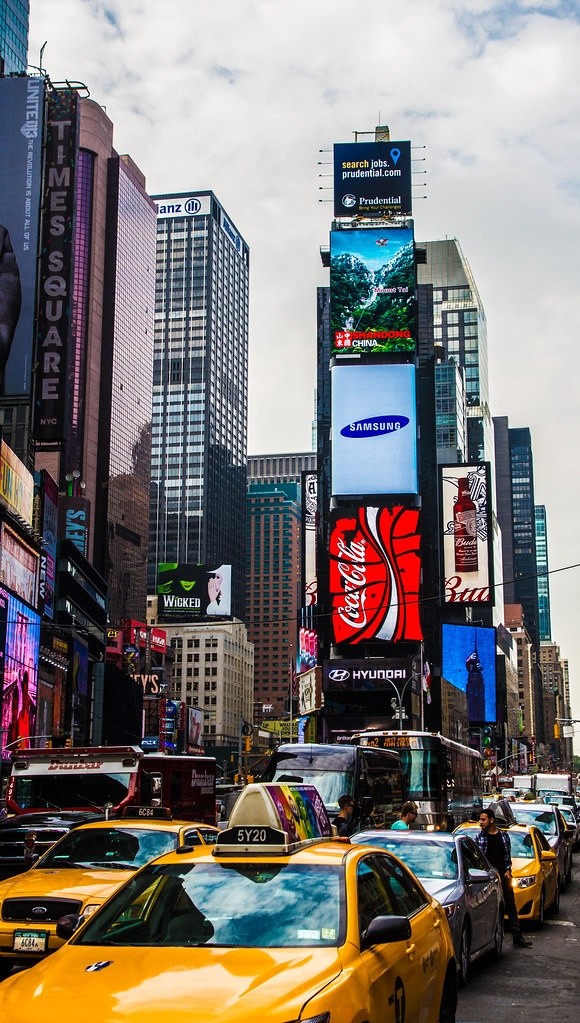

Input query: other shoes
[512,937,533,947]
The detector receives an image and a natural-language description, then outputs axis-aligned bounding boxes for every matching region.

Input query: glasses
[408,811,417,817]
[343,804,355,808]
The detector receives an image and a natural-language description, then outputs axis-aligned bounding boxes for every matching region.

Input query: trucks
[510,773,573,795]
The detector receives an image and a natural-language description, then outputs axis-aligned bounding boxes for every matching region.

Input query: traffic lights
[247,775,254,783]
[233,774,241,782]
[245,738,253,751]
[482,728,494,769]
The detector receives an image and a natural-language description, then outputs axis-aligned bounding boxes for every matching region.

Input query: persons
[157,563,231,615]
[390,802,418,831]
[331,795,354,836]
[473,809,533,947]
[6,671,30,752]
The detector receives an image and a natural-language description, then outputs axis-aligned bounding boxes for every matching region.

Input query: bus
[216,777,231,784]
[351,729,483,830]
[259,742,407,841]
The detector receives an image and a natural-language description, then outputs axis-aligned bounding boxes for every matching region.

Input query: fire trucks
[5,747,220,826]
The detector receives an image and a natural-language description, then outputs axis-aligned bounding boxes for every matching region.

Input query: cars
[544,791,560,796]
[0,785,465,1023]
[501,788,523,799]
[349,828,506,986]
[451,820,561,931]
[543,796,578,814]
[520,788,534,799]
[509,802,573,893]
[1,811,114,881]
[0,805,221,979]
[556,804,580,850]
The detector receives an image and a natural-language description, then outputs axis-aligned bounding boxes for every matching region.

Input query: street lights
[497,750,576,770]
[369,673,422,730]
